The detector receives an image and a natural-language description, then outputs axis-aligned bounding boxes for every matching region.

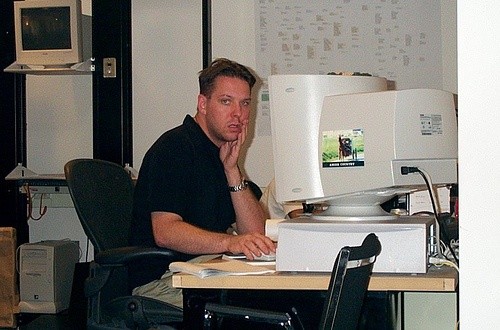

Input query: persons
[128,57,277,330]
[231,175,325,235]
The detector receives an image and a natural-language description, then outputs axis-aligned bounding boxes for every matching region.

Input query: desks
[171,258,459,330]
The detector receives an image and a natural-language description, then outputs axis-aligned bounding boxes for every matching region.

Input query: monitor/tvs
[267,75,458,221]
[14,0,92,70]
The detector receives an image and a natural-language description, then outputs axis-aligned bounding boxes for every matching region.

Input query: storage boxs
[0,227,19,329]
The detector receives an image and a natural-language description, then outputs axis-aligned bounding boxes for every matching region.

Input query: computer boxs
[20,239,79,314]
[275,216,436,276]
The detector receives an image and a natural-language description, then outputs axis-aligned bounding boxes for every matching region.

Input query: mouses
[246,249,275,261]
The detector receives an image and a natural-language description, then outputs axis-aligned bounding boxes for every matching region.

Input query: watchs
[229,178,248,192]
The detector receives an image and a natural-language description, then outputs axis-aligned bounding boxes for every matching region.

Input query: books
[168,259,276,278]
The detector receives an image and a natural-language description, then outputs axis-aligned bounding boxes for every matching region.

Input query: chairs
[202,233,381,330]
[65,158,190,330]
